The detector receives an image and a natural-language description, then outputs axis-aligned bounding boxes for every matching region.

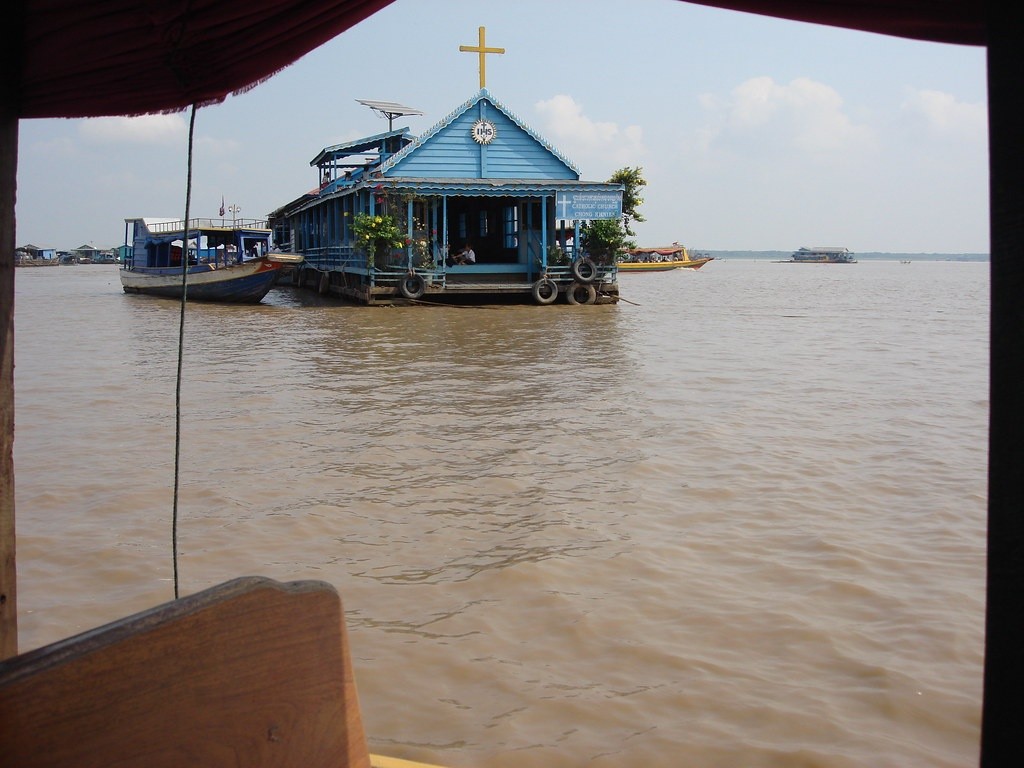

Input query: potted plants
[536,245,571,284]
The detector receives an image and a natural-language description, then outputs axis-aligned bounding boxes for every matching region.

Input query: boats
[791,246,858,264]
[616,242,713,272]
[118,195,304,306]
[901,259,911,263]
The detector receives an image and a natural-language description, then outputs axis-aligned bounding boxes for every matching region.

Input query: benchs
[0,579,445,768]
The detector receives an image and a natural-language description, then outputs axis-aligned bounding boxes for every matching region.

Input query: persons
[437,242,476,268]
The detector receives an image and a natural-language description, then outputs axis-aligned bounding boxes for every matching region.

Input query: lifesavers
[533,278,559,304]
[400,272,426,300]
[566,280,599,305]
[573,257,597,282]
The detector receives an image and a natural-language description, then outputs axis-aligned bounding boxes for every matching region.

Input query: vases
[376,243,386,269]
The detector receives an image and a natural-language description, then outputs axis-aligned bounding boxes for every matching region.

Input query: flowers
[344,212,409,269]
